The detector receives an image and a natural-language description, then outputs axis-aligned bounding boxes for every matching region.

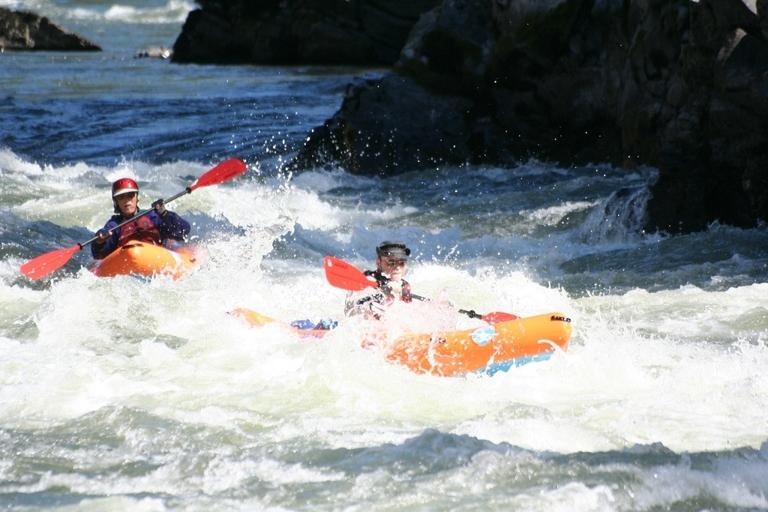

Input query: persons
[345,241,413,319]
[91,177,191,260]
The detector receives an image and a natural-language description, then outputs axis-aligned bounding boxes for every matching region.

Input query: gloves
[96,229,112,245]
[152,199,166,215]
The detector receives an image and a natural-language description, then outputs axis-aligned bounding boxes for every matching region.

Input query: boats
[227,304,571,376]
[89,236,199,282]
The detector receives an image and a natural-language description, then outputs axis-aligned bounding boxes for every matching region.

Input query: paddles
[20,159,248,281]
[324,255,520,325]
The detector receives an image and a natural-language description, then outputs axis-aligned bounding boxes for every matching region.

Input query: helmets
[111,178,139,196]
[376,241,410,260]
